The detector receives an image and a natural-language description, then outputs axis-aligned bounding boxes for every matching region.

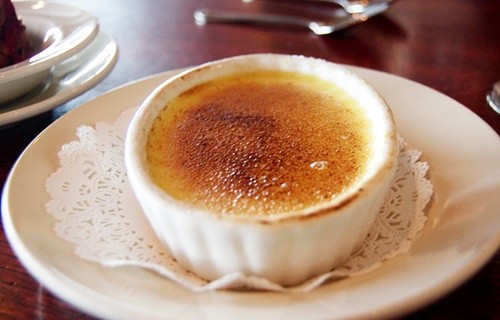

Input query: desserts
[127,51,400,289]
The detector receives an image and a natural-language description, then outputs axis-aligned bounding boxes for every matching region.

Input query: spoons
[192,0,390,35]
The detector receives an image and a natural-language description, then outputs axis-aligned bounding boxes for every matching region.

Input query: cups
[126,46,397,288]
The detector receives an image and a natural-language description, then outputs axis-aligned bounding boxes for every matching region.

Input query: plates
[0,25,122,134]
[1,0,101,108]
[1,54,500,320]
